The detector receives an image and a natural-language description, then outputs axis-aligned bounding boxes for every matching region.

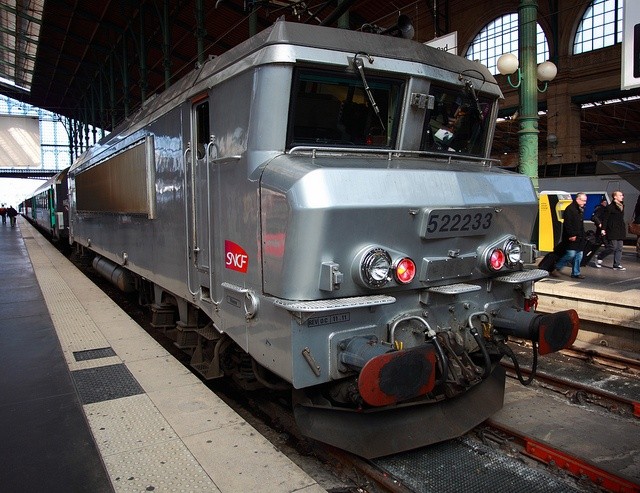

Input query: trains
[17,12,581,466]
[493,153,640,243]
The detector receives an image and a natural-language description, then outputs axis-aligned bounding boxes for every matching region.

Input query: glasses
[579,198,587,202]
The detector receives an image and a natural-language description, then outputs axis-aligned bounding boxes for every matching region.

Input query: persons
[593,191,627,271]
[7,206,17,226]
[590,199,608,248]
[550,193,587,278]
[0,204,7,224]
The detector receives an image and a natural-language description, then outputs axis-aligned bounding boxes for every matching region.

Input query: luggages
[2,216,6,223]
[538,252,557,271]
[569,234,603,267]
[595,233,600,242]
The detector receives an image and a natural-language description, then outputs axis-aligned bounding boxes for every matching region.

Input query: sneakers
[594,254,603,268]
[571,274,584,279]
[551,269,562,277]
[613,265,626,271]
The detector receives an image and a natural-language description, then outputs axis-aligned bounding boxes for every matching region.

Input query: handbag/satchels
[556,241,567,256]
[584,230,596,248]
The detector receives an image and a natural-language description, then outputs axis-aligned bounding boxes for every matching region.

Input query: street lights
[496,51,557,201]
